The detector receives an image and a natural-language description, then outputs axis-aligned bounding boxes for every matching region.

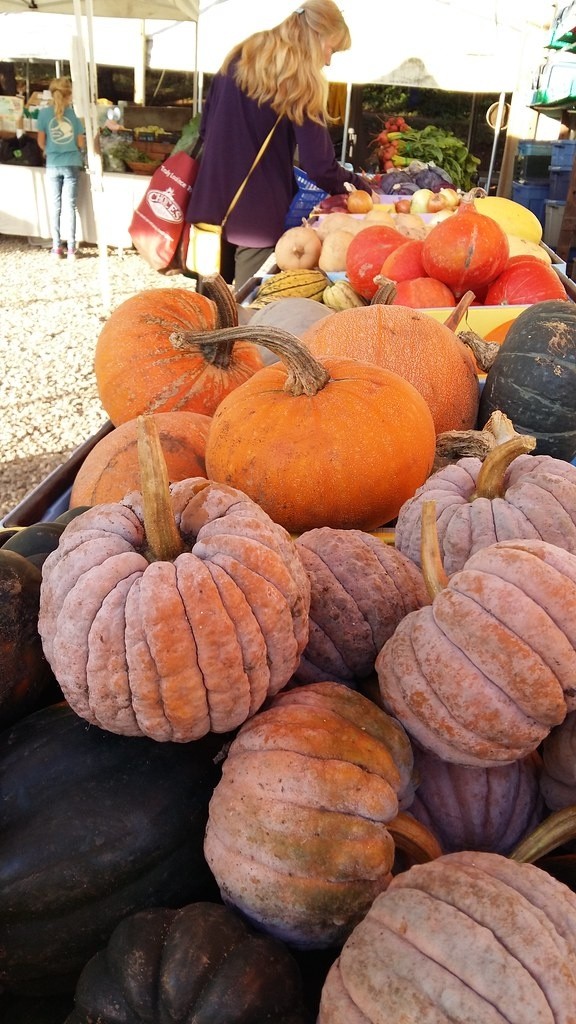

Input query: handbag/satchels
[176,220,223,278]
[128,136,204,278]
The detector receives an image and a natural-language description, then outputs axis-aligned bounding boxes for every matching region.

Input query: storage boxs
[0,215,576,1024]
[550,139,576,166]
[521,143,551,157]
[22,118,38,131]
[311,173,412,215]
[548,167,572,200]
[523,155,551,181]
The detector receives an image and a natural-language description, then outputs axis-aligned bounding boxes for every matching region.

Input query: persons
[35,77,86,260]
[185,1,380,292]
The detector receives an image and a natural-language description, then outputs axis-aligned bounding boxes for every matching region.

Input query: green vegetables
[110,142,155,163]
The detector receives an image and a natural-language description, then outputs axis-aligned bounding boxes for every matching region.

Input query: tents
[0,0,198,117]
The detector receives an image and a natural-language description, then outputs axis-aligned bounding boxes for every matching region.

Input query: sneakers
[51,248,64,259]
[67,249,85,259]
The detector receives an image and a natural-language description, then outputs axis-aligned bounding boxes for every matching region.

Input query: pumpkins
[241,160,567,311]
[0,275,576,1024]
